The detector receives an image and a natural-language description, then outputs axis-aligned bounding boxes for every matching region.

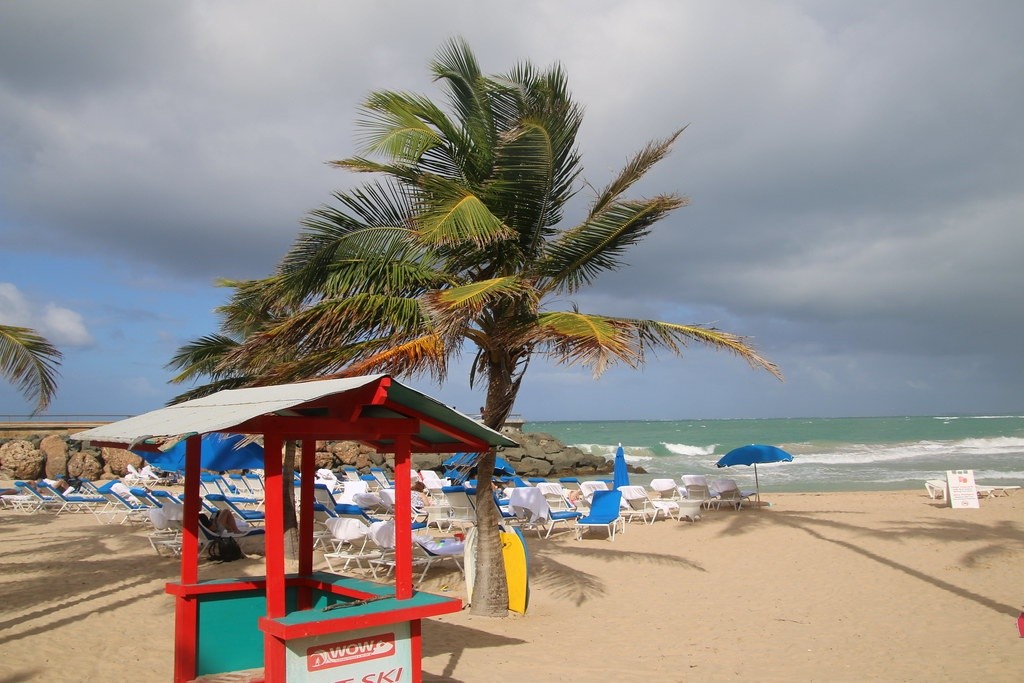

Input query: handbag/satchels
[217,536,242,561]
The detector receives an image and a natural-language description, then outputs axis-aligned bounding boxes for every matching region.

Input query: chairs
[0,472,759,593]
[925,479,1021,504]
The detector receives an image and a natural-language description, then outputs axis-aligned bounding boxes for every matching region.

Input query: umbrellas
[715,444,794,502]
[128,433,264,472]
[442,453,516,477]
[612,442,629,491]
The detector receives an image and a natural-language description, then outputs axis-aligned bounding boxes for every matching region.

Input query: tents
[68,374,519,682]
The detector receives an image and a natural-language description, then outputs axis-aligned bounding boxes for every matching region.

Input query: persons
[411,480,429,513]
[480,407,485,420]
[198,497,247,535]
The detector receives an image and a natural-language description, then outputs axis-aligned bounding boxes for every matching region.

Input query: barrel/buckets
[454,534,464,541]
